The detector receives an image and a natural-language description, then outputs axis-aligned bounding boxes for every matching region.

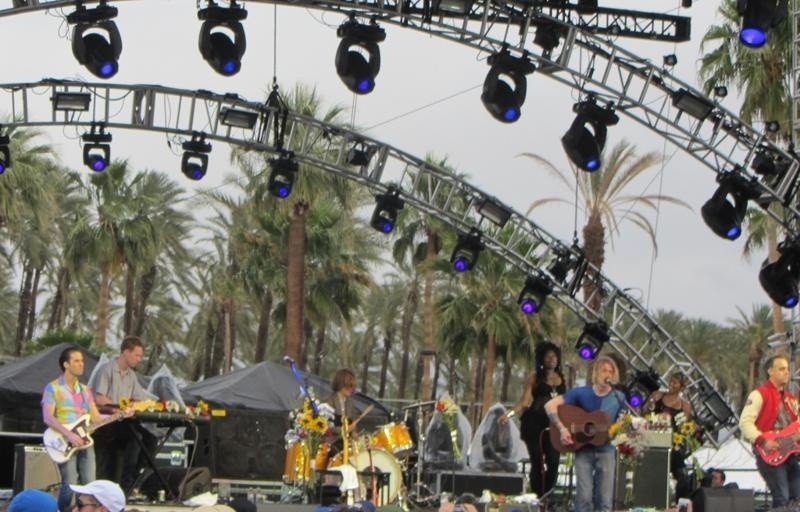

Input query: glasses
[78,499,102,509]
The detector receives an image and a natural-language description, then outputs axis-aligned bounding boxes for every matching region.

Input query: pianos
[99,404,211,426]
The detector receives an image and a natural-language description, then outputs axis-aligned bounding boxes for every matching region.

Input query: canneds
[157,489,166,502]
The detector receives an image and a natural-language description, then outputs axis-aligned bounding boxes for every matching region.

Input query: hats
[192,499,257,512]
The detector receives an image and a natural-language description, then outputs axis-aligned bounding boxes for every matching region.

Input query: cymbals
[403,400,435,410]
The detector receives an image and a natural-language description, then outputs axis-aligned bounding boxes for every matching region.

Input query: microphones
[606,377,630,394]
[283,355,295,363]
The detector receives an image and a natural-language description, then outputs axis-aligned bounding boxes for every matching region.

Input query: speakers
[12,443,62,493]
[614,444,670,512]
[435,470,525,500]
[689,487,756,512]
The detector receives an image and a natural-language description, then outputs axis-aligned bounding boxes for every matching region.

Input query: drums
[324,447,402,504]
[373,421,414,458]
[282,434,331,485]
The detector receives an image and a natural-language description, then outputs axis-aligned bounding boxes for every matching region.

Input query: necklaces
[544,370,562,400]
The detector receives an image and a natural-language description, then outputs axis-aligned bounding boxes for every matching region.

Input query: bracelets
[650,397,653,401]
[504,405,518,420]
[558,426,569,434]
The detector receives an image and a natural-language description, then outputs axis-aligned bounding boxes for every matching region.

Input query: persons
[89,336,164,505]
[637,369,693,506]
[737,354,799,512]
[494,340,567,499]
[684,467,725,512]
[320,368,374,441]
[39,346,134,511]
[230,499,257,510]
[319,486,480,510]
[542,356,650,511]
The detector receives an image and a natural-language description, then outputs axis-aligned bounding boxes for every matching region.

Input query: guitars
[755,420,800,466]
[43,411,126,463]
[549,404,611,452]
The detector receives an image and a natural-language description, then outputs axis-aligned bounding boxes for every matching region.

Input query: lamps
[700,162,799,311]
[367,183,488,274]
[733,0,790,50]
[79,119,300,199]
[515,274,662,409]
[66,2,620,176]
